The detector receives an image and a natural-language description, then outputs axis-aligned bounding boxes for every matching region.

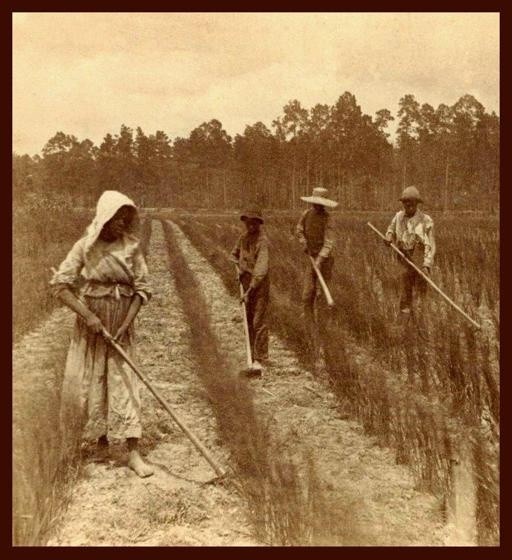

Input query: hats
[397,185,425,203]
[240,204,264,224]
[300,187,338,208]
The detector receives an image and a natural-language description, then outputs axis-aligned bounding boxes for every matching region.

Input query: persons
[382,185,437,315]
[228,203,273,378]
[295,186,337,321]
[49,189,156,481]
